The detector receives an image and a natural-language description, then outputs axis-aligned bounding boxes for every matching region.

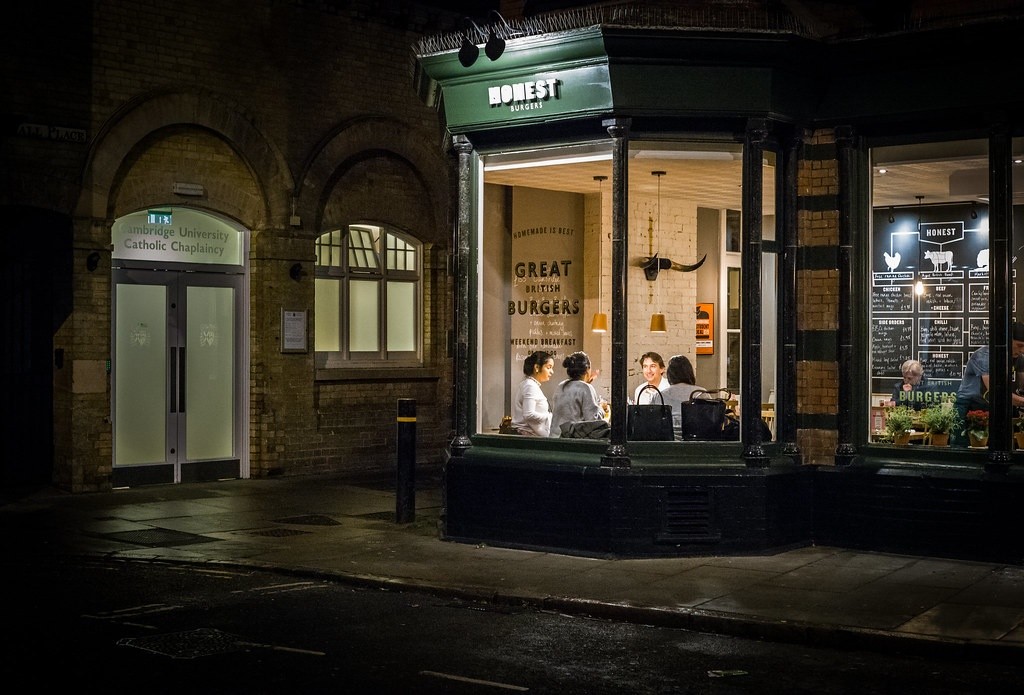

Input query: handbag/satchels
[681,387,731,439]
[625,384,674,440]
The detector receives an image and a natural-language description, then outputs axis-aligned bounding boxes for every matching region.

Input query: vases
[968,433,988,447]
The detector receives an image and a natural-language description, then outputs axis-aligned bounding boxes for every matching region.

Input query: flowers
[961,410,990,440]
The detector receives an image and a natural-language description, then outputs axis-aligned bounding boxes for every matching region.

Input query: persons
[656,355,712,439]
[959,322,1024,419]
[550,352,608,438]
[635,352,669,404]
[512,351,554,435]
[883,359,934,431]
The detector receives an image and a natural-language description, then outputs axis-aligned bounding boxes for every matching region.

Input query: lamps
[914,196,924,295]
[591,175,608,333]
[458,16,490,68]
[650,170,667,333]
[485,9,522,61]
[888,207,895,223]
[969,201,978,219]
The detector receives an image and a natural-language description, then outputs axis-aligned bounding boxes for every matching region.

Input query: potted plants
[1012,411,1024,449]
[882,398,964,447]
